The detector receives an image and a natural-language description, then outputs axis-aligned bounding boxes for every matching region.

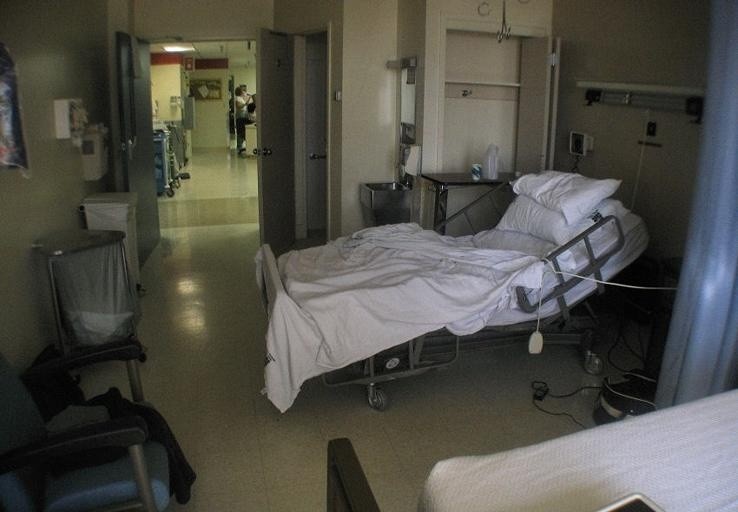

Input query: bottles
[482,143,499,180]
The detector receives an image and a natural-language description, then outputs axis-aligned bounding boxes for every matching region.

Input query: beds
[255,171,649,413]
[325,391,737,512]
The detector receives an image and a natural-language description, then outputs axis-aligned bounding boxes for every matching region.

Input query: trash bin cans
[41,229,142,344]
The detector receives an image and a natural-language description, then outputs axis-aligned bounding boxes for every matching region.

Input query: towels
[404,145,423,176]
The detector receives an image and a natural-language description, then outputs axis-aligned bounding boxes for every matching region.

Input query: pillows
[513,171,622,221]
[497,196,630,247]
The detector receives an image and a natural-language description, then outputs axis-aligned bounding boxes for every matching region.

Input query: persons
[239,85,256,124]
[235,87,250,152]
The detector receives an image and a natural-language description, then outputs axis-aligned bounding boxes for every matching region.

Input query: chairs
[1,340,172,511]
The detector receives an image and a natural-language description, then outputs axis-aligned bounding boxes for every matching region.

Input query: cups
[471,163,481,181]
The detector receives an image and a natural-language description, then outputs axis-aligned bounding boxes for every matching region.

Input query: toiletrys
[471,163,483,181]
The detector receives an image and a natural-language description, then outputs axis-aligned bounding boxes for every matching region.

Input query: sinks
[360,181,408,209]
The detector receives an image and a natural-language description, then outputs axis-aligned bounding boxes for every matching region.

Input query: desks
[418,172,519,235]
